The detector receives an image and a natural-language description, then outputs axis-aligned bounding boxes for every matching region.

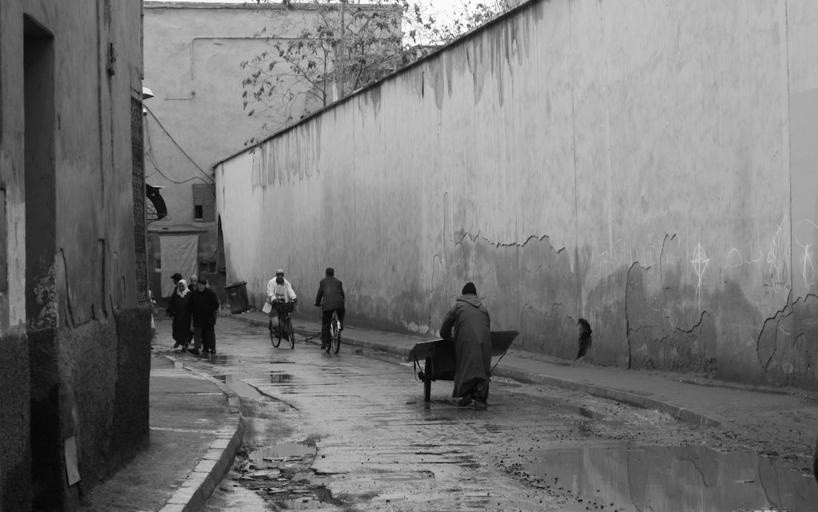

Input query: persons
[149,289,155,349]
[265,269,298,333]
[314,267,346,348]
[165,273,219,355]
[439,282,492,407]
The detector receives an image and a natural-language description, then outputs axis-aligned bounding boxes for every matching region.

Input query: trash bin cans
[224,281,248,314]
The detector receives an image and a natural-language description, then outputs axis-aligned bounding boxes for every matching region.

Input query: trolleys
[411,337,496,406]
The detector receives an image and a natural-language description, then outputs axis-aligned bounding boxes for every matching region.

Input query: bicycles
[313,301,347,354]
[264,297,303,351]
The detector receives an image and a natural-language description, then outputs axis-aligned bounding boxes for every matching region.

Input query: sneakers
[458,398,488,409]
[174,341,216,354]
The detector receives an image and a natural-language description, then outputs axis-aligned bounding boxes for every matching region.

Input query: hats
[463,282,477,295]
